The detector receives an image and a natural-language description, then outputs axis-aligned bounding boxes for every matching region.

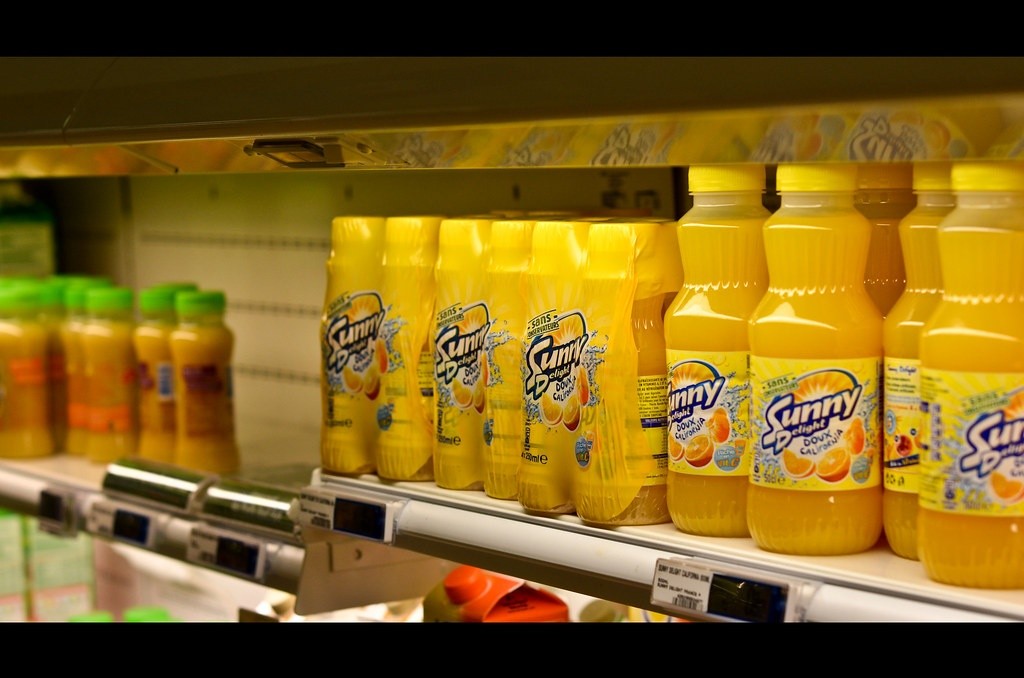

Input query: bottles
[316,161,1024,623]
[0,272,241,478]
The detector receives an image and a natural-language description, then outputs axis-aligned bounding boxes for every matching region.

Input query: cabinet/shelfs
[2,54,1023,622]
